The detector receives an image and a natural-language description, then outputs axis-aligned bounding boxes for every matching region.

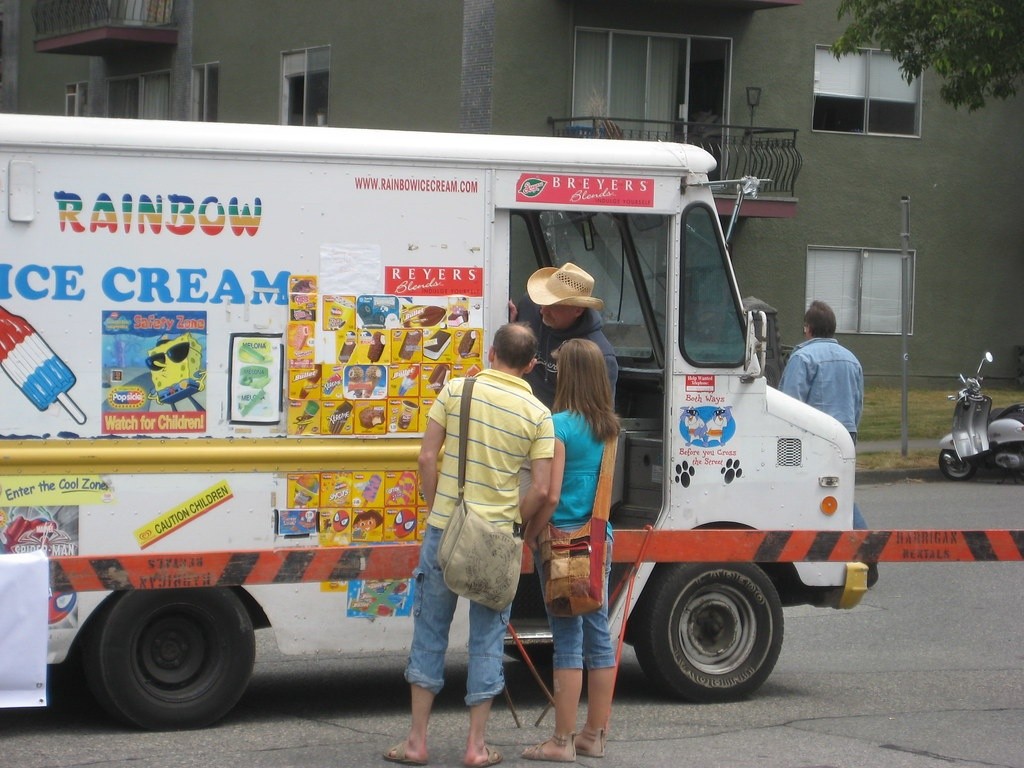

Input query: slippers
[383,743,427,766]
[473,745,503,768]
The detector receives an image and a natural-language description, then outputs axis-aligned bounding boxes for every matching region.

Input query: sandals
[576,725,607,757]
[521,731,577,762]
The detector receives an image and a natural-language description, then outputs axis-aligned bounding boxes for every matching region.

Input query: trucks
[0,115,882,733]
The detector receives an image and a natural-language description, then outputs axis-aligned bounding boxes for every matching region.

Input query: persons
[383,325,555,768]
[507,263,618,497]
[778,300,879,589]
[519,338,620,763]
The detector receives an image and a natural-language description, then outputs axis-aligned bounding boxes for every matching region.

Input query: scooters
[939,351,1024,485]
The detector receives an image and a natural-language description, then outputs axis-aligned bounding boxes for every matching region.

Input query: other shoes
[868,562,879,588]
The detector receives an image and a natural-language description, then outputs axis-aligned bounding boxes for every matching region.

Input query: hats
[527,263,604,310]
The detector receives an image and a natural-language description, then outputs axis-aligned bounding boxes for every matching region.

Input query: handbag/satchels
[437,498,523,611]
[538,518,609,615]
